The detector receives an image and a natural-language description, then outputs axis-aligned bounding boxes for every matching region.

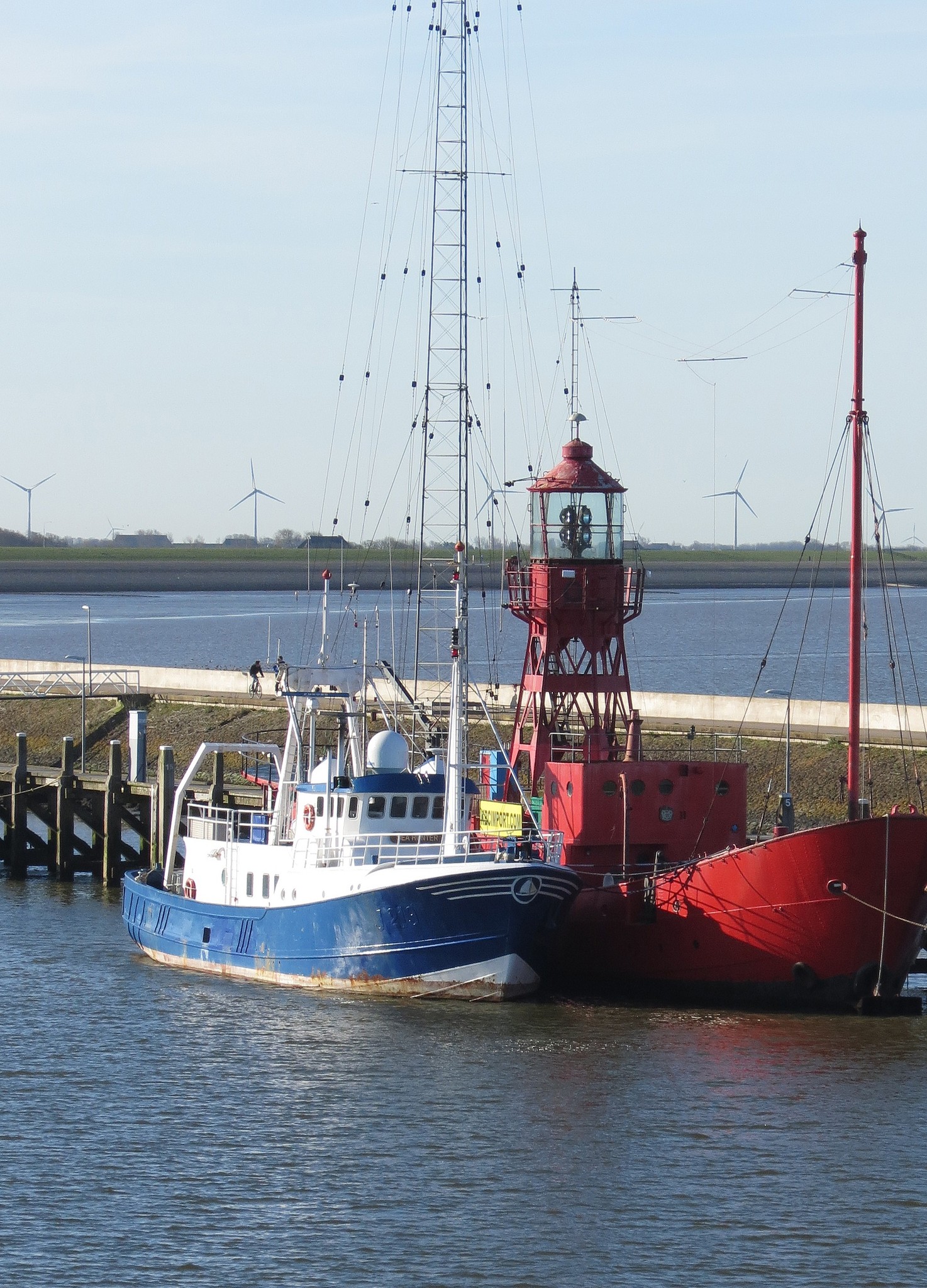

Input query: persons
[273,656,287,697]
[248,660,265,699]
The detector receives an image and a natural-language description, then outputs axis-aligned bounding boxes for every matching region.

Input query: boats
[465,221,927,1008]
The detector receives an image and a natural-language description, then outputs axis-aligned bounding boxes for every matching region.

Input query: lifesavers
[302,803,315,831]
[184,878,196,900]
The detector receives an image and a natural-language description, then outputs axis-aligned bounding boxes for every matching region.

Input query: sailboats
[121,1,589,1006]
